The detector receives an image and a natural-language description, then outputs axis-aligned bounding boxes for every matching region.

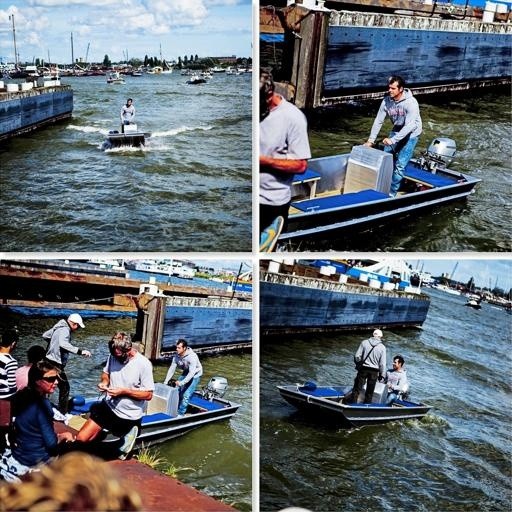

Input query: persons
[75,331,155,444]
[259,69,312,234]
[42,313,91,416]
[120,98,136,134]
[362,76,423,198]
[9,360,139,469]
[381,354,410,406]
[15,344,48,400]
[163,338,203,416]
[0,330,18,456]
[351,328,387,404]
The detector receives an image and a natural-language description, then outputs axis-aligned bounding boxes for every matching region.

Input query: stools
[140,410,172,426]
[187,394,224,412]
[392,400,417,409]
[306,389,345,404]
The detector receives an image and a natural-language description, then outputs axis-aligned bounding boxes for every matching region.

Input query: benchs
[402,165,460,188]
[289,189,394,212]
[284,168,321,202]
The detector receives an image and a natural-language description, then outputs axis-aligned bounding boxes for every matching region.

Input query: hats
[373,330,384,337]
[68,313,85,328]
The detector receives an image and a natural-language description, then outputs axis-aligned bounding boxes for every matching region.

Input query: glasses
[43,375,57,383]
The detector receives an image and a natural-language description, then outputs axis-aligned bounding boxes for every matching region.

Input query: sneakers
[118,426,138,461]
[260,216,284,252]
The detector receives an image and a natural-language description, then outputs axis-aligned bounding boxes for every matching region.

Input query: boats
[274,380,434,427]
[1,54,252,84]
[48,259,198,280]
[98,120,150,151]
[271,134,482,233]
[61,379,241,452]
[413,269,512,311]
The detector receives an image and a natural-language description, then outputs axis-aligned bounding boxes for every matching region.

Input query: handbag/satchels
[0,400,11,427]
[355,360,365,370]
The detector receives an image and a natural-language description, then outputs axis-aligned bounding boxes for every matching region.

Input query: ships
[259,260,428,338]
